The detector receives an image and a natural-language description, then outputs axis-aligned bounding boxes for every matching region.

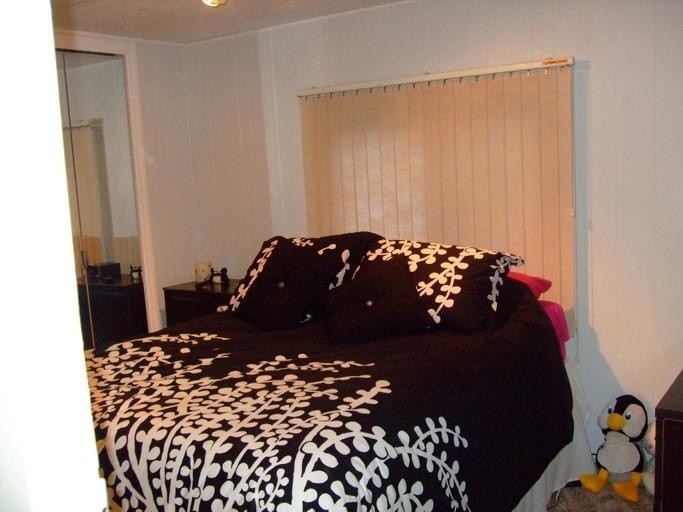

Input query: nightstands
[655,369,683,512]
[77,273,148,349]
[163,277,243,326]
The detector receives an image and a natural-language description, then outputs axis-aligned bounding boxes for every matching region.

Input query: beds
[84,303,584,512]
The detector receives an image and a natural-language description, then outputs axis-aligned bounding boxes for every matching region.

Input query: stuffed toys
[578,393,649,503]
[639,418,654,497]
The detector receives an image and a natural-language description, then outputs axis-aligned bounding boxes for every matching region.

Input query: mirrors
[54,28,161,349]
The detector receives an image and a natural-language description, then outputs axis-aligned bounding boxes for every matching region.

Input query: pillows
[229,235,341,331]
[508,270,552,302]
[345,238,524,332]
[288,232,383,327]
[314,258,421,333]
[481,275,529,332]
